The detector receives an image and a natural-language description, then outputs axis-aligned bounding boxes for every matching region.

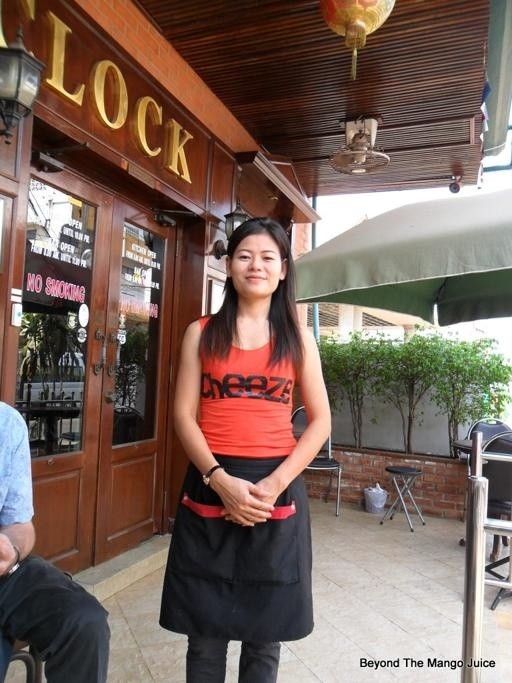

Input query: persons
[158,217,332,681]
[0,398,111,682]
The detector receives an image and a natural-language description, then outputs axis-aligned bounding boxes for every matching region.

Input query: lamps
[446,172,465,193]
[214,193,250,260]
[1,20,45,146]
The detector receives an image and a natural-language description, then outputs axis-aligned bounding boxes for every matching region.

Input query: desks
[28,404,81,457]
[447,435,512,564]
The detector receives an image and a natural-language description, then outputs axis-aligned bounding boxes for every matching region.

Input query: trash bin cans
[364,488,387,514]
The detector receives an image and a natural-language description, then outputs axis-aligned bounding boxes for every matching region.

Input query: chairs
[458,414,511,523]
[477,428,512,611]
[290,401,347,519]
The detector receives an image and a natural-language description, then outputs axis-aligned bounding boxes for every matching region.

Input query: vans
[21,350,85,417]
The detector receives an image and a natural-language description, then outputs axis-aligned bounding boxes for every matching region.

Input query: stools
[5,631,45,681]
[57,429,82,453]
[29,438,46,456]
[377,461,429,531]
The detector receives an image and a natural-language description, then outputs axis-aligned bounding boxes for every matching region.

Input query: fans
[330,111,391,176]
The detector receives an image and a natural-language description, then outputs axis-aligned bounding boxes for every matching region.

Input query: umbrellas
[289,190,511,327]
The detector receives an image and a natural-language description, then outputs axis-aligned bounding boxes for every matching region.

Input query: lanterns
[316,0,398,80]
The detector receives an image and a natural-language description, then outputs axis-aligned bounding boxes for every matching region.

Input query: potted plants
[112,322,151,444]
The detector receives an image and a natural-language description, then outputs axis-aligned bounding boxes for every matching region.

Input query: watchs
[6,547,21,576]
[202,465,222,486]
